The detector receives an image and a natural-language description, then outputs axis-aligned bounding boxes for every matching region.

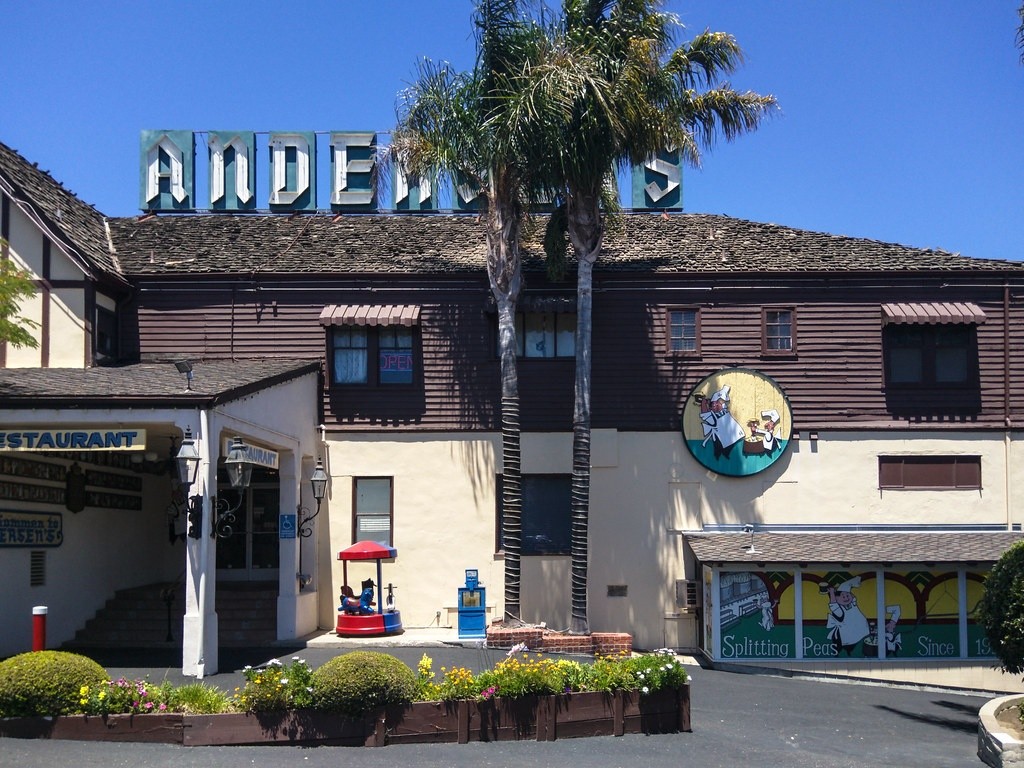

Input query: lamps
[175,359,195,392]
[297,454,328,539]
[210,432,255,541]
[175,424,204,540]
